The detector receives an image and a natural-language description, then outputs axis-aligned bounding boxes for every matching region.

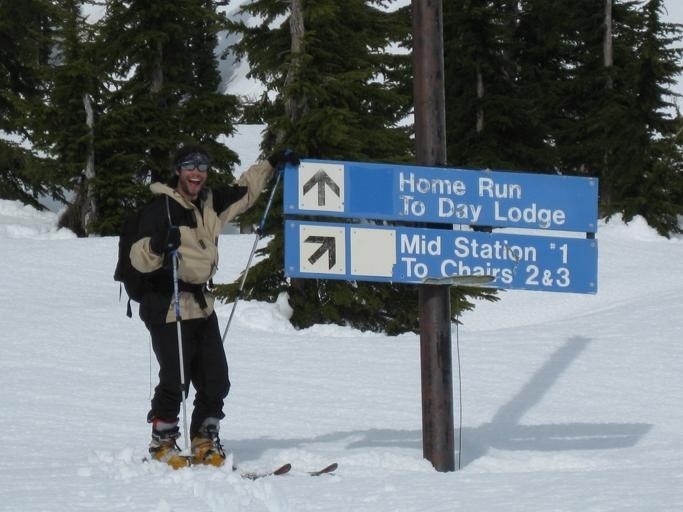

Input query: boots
[189,410,226,467]
[148,418,191,470]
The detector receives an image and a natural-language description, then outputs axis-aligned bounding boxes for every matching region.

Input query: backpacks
[114,195,173,303]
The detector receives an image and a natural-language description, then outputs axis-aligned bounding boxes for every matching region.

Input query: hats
[174,143,209,161]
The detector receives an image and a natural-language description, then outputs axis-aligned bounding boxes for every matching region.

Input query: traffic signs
[281,155,605,297]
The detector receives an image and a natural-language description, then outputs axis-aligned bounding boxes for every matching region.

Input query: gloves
[267,149,300,170]
[150,224,181,255]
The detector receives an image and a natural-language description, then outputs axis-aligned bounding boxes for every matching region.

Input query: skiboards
[139,454,338,480]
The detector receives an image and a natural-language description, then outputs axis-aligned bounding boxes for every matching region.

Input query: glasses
[178,160,210,172]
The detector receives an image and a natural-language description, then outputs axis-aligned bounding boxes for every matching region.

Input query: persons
[113,141,306,469]
